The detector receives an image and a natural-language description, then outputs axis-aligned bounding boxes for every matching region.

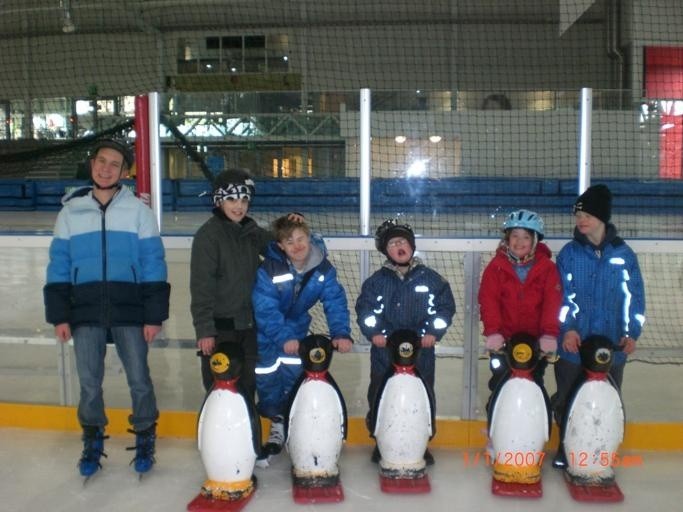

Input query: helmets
[500,209,545,241]
[88,132,134,168]
[212,169,255,205]
[375,216,415,252]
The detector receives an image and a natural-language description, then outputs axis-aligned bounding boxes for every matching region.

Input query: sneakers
[256,447,267,460]
[263,416,286,455]
[372,446,380,464]
[552,450,566,469]
[423,449,434,466]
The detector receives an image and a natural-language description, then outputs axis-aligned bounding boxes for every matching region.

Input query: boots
[80,426,105,475]
[135,424,156,473]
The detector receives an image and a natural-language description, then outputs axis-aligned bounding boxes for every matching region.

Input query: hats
[573,184,611,222]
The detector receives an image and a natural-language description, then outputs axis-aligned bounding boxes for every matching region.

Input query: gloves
[485,333,504,352]
[539,335,557,352]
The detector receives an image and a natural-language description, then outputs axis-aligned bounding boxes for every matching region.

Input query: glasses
[386,237,407,247]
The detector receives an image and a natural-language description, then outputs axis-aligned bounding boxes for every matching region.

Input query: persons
[44,138,170,477]
[551,185,646,471]
[190,169,307,468]
[482,94,510,110]
[251,216,354,454]
[478,209,563,369]
[355,220,456,464]
